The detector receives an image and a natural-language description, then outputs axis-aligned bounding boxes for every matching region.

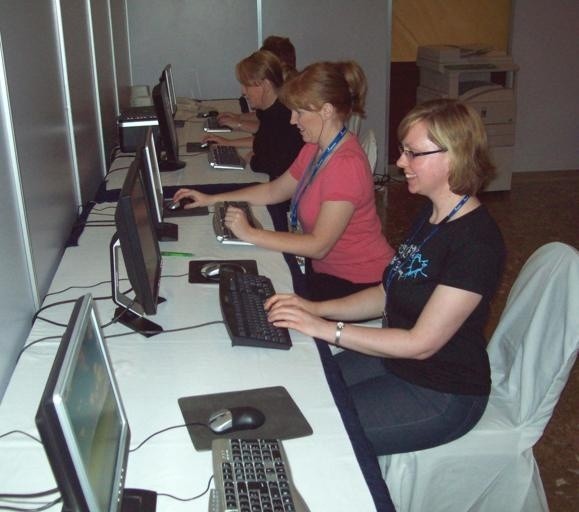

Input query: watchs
[335,322,345,346]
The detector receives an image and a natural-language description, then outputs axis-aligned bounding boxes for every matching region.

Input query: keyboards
[212,437,310,512]
[213,201,263,245]
[219,272,293,350]
[204,117,232,133]
[208,144,246,170]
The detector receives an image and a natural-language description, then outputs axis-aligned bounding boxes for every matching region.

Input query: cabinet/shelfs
[416,44,521,192]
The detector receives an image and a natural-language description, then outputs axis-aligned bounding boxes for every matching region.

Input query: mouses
[200,141,217,149]
[202,111,219,118]
[207,406,265,435]
[166,198,195,211]
[201,261,247,282]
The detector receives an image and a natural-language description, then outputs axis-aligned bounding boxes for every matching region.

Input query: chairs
[376,241,579,512]
[357,129,375,175]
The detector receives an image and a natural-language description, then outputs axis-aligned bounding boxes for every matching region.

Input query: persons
[264,98,506,457]
[198,50,304,232]
[172,60,397,324]
[215,36,299,132]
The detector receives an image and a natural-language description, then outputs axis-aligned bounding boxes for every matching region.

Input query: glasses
[398,145,444,161]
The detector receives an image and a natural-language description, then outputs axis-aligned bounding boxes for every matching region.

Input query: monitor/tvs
[162,64,184,127]
[136,126,179,241]
[152,80,185,172]
[35,293,157,511]
[109,160,167,338]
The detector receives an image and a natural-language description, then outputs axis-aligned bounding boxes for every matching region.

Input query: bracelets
[237,120,242,130]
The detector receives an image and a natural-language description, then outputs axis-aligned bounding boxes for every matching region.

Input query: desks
[34,98,396,512]
